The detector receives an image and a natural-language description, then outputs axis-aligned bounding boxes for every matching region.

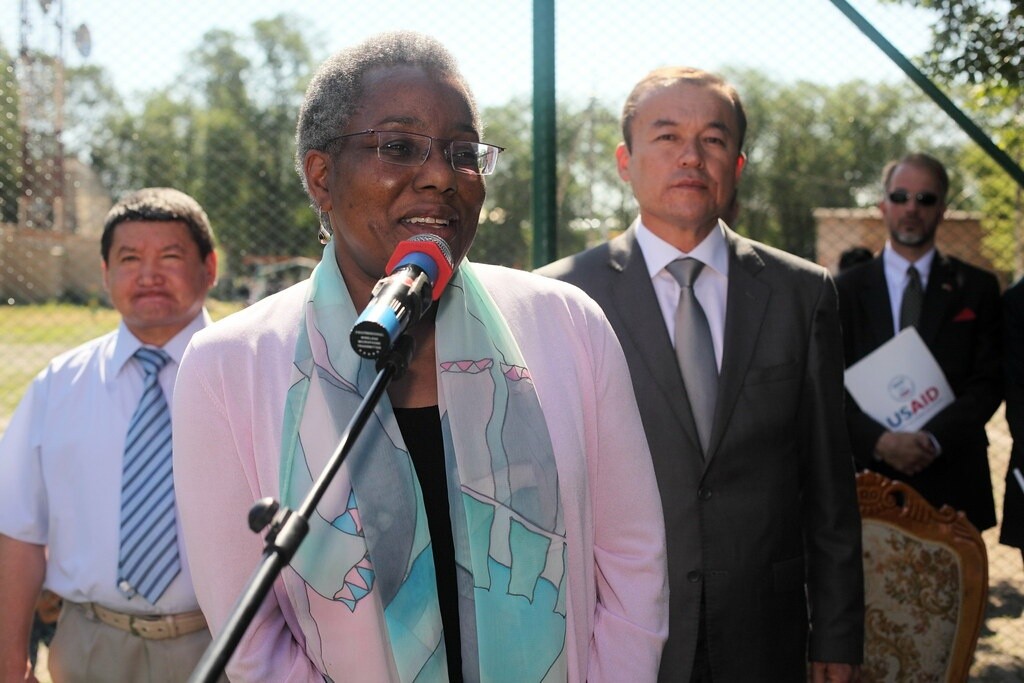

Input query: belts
[93,605,207,641]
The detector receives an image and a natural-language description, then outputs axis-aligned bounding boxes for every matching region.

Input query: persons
[997,276,1024,551]
[0,186,221,683]
[532,67,867,683]
[837,246,873,278]
[835,152,1012,534]
[171,32,669,683]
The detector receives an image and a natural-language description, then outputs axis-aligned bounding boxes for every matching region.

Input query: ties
[899,266,925,336]
[117,343,184,604]
[664,257,719,460]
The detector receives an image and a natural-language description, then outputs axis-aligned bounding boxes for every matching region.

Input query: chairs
[804,468,991,683]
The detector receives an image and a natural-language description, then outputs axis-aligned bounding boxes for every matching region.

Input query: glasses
[316,129,507,175]
[888,192,941,208]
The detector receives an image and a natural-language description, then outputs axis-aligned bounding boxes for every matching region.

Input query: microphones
[350,233,453,359]
